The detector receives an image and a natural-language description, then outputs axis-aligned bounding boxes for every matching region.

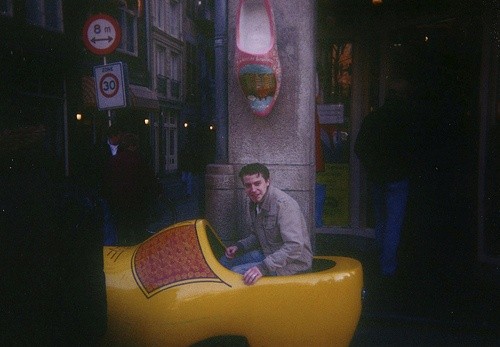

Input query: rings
[252,275,256,277]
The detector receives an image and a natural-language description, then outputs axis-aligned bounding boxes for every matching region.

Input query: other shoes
[233,1,281,116]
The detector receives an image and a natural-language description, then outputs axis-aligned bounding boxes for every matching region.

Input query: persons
[352,77,424,281]
[97,125,137,252]
[216,162,312,288]
